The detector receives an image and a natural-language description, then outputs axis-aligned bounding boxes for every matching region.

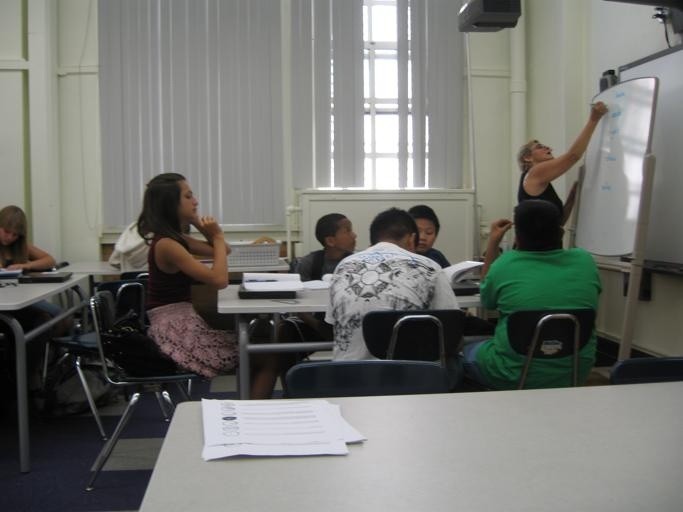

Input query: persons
[107,217,150,275]
[326,207,460,362]
[0,204,71,363]
[137,172,286,399]
[450,199,603,391]
[274,213,358,396]
[517,101,610,227]
[407,206,494,337]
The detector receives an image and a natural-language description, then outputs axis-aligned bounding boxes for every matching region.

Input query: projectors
[460,2,520,32]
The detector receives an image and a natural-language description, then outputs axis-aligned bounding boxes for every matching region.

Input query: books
[442,260,484,285]
[242,272,304,293]
[303,273,332,290]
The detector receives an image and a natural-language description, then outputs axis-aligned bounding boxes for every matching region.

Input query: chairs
[361,310,467,371]
[99,279,129,292]
[508,308,598,391]
[34,282,147,443]
[140,274,148,285]
[54,261,70,270]
[287,362,444,396]
[608,356,683,384]
[86,291,198,493]
[122,270,149,279]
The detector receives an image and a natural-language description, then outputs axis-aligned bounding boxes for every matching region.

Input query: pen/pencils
[246,279,278,283]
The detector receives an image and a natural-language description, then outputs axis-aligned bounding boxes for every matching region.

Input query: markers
[589,103,608,107]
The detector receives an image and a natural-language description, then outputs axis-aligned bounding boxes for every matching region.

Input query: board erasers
[619,256,632,262]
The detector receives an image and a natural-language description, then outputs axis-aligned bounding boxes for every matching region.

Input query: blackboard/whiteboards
[573,76,659,258]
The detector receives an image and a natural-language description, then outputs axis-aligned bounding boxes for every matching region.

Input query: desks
[2,273,92,475]
[219,281,482,397]
[137,383,680,512]
[62,253,291,296]
